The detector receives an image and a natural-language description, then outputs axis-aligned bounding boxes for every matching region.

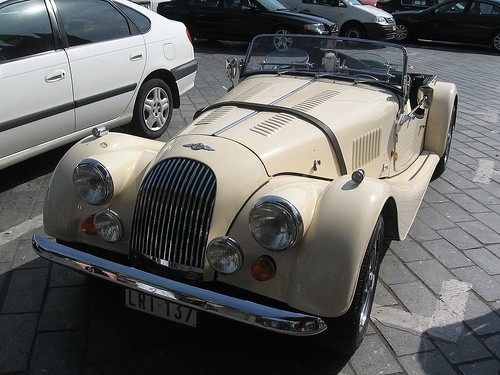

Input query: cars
[32,33,463,357]
[155,0,339,54]
[388,0,500,54]
[0,0,197,177]
[279,0,396,43]
[375,0,442,16]
